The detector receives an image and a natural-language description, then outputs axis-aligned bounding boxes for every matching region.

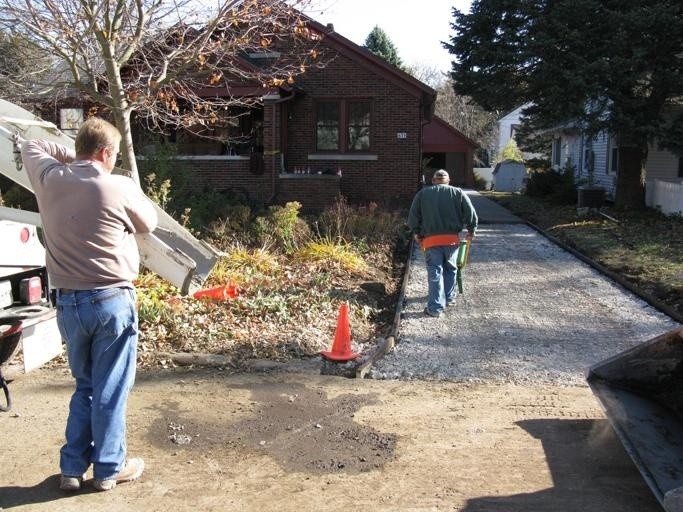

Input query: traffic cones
[318,300,364,362]
[191,283,240,302]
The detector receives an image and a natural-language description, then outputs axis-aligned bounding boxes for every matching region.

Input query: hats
[434,169,449,178]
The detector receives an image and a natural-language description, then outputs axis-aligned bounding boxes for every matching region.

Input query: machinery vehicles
[0,95,225,417]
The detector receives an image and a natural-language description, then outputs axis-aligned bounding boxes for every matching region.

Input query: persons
[405,167,479,317]
[14,112,161,496]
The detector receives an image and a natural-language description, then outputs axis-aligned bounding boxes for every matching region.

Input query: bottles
[293,165,311,174]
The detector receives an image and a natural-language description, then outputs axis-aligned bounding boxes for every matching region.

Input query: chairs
[0,316,24,412]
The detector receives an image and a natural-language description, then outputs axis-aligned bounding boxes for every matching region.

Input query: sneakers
[424,299,456,317]
[92,457,144,490]
[59,473,86,490]
